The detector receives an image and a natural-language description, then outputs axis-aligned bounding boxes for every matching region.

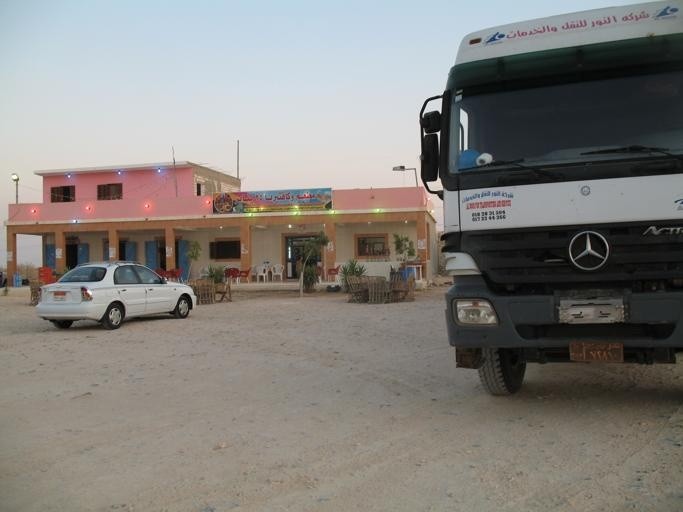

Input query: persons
[294,255,304,278]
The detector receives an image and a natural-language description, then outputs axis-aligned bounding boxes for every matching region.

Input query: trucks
[419,0,683,397]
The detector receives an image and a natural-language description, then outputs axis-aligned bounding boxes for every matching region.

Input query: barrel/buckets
[38,267,52,285]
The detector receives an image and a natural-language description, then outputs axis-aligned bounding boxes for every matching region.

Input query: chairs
[346,270,409,304]
[155,267,232,305]
[222,262,284,285]
[327,263,341,284]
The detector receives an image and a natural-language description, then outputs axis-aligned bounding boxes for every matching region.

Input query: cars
[35,260,197,330]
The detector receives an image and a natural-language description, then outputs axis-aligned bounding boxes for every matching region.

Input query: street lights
[11,172,19,203]
[392,165,418,187]
